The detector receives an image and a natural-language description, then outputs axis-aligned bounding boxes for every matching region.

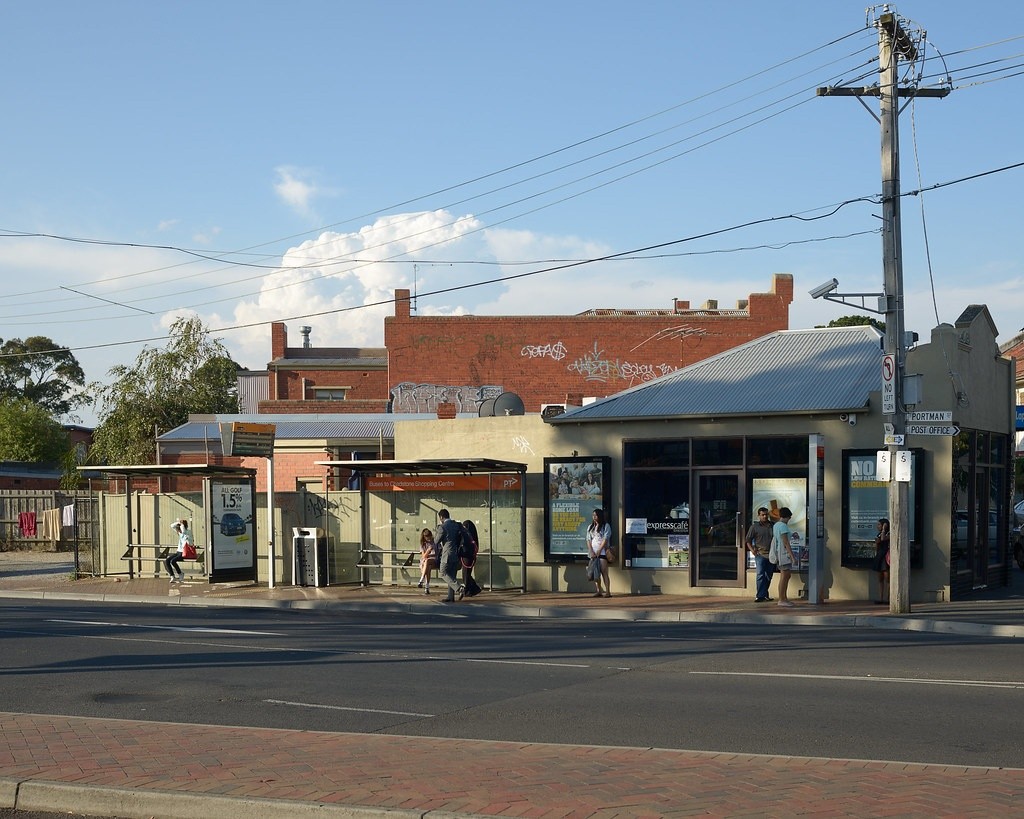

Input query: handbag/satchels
[587,557,600,582]
[182,543,197,559]
[769,537,777,564]
[605,548,616,563]
[886,548,890,565]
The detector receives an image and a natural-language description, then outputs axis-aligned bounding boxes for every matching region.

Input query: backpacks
[457,522,475,560]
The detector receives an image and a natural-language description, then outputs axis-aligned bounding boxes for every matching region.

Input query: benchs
[121,543,206,578]
[357,548,523,589]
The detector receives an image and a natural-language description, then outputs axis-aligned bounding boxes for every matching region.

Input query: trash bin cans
[292,527,335,586]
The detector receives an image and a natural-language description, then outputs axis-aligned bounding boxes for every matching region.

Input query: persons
[558,462,601,496]
[434,509,466,602]
[873,518,890,603]
[461,520,482,597]
[770,508,796,607]
[417,528,441,595]
[586,509,611,598]
[746,508,775,603]
[164,518,194,583]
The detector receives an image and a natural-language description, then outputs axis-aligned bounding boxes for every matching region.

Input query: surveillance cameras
[840,414,849,421]
[808,277,838,299]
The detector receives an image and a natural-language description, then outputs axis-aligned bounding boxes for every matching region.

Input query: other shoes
[418,582,423,588]
[885,601,889,605]
[593,593,602,597]
[777,600,795,607]
[458,586,465,600]
[425,588,429,594]
[754,597,763,602]
[442,597,454,602]
[463,594,472,597]
[764,596,774,601]
[473,588,481,595]
[604,593,610,598]
[170,575,175,583]
[178,572,184,582]
[874,600,883,604]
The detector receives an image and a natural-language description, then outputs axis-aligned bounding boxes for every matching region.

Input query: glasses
[788,516,791,519]
